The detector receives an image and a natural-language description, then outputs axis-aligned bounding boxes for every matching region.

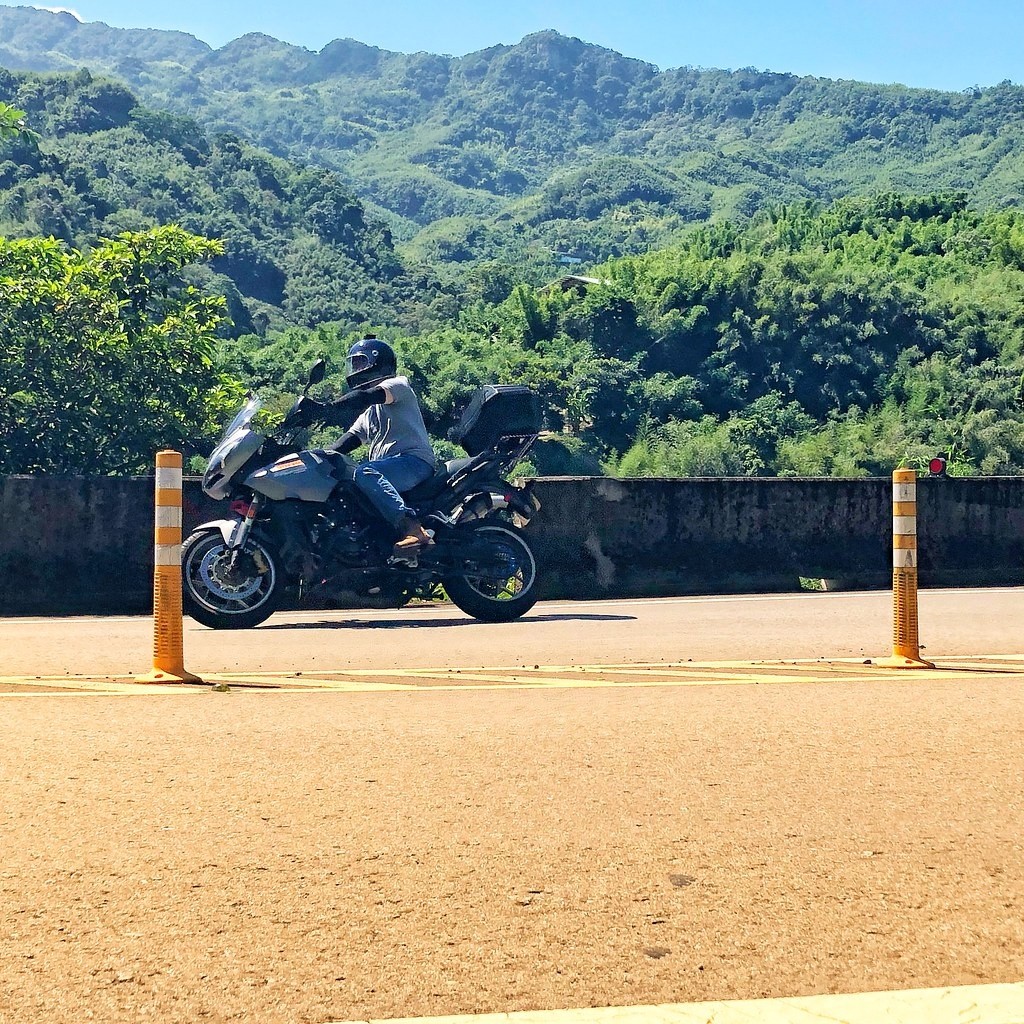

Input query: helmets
[346,339,397,390]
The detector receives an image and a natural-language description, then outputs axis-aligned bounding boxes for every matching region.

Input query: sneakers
[394,520,434,556]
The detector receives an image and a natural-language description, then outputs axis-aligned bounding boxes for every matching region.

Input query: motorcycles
[180,358,549,629]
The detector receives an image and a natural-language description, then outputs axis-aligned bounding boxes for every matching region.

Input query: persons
[285,339,437,558]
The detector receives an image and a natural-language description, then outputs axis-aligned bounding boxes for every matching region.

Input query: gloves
[285,395,333,426]
